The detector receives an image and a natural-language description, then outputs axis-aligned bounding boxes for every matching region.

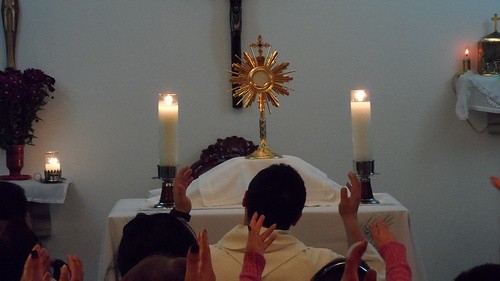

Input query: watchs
[170,207,190,222]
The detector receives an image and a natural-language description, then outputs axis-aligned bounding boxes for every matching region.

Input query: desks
[97,191,427,281]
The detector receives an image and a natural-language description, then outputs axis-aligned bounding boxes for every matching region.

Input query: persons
[110,165,414,281]
[0,181,84,281]
[454,263,500,281]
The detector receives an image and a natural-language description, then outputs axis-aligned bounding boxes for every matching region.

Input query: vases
[0,144,32,180]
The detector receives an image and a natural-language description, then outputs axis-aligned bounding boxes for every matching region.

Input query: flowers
[0,67,56,146]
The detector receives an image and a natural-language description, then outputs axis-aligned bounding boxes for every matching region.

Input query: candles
[463,49,470,72]
[159,94,180,165]
[351,89,373,161]
[46,152,61,172]
